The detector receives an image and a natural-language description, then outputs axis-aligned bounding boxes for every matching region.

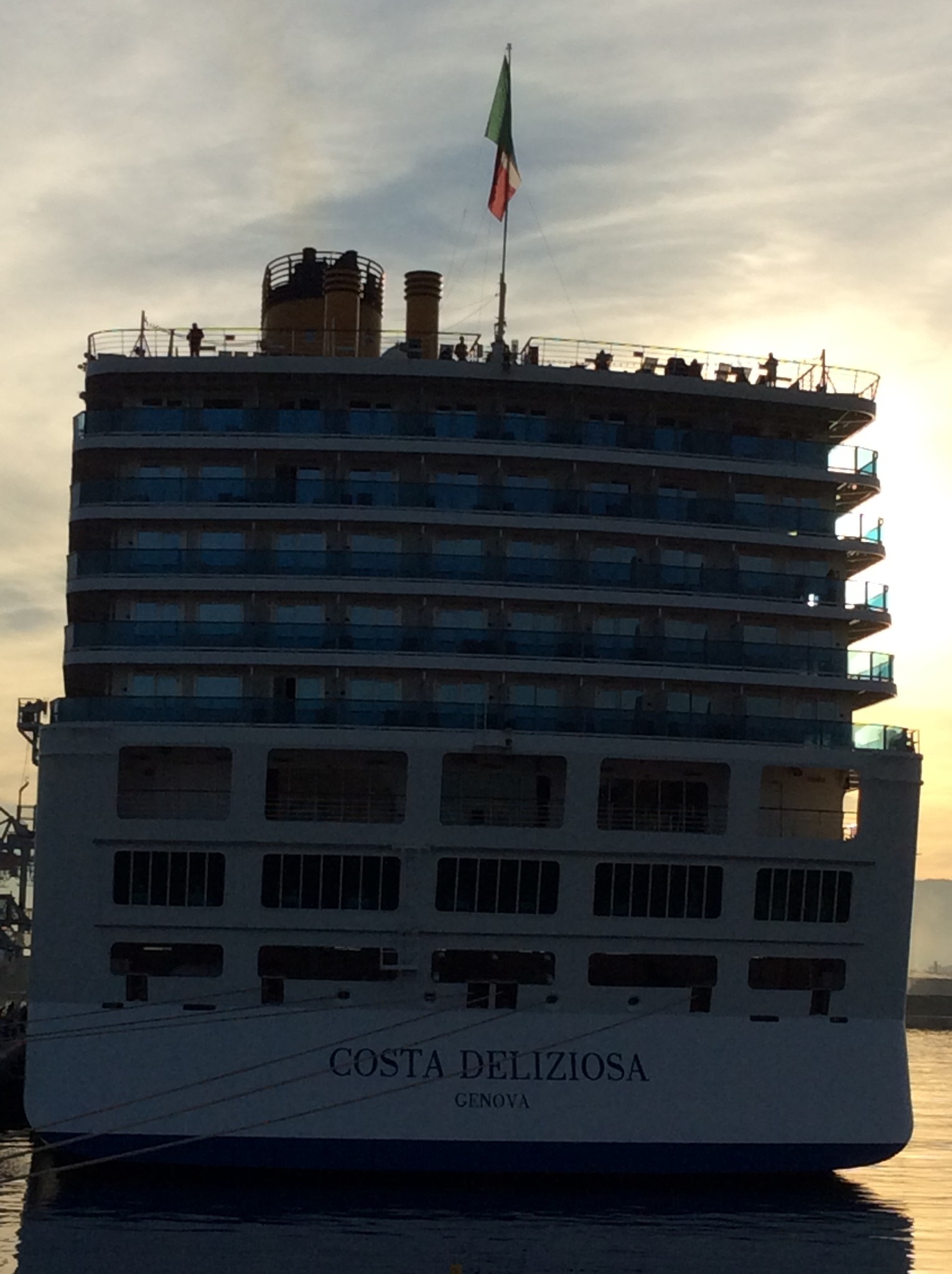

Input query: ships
[0,36,928,1184]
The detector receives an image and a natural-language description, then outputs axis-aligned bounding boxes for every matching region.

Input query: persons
[765,352,779,388]
[595,350,609,371]
[186,322,204,357]
[454,336,468,363]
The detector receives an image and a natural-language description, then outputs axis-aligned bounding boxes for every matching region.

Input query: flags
[483,55,523,223]
